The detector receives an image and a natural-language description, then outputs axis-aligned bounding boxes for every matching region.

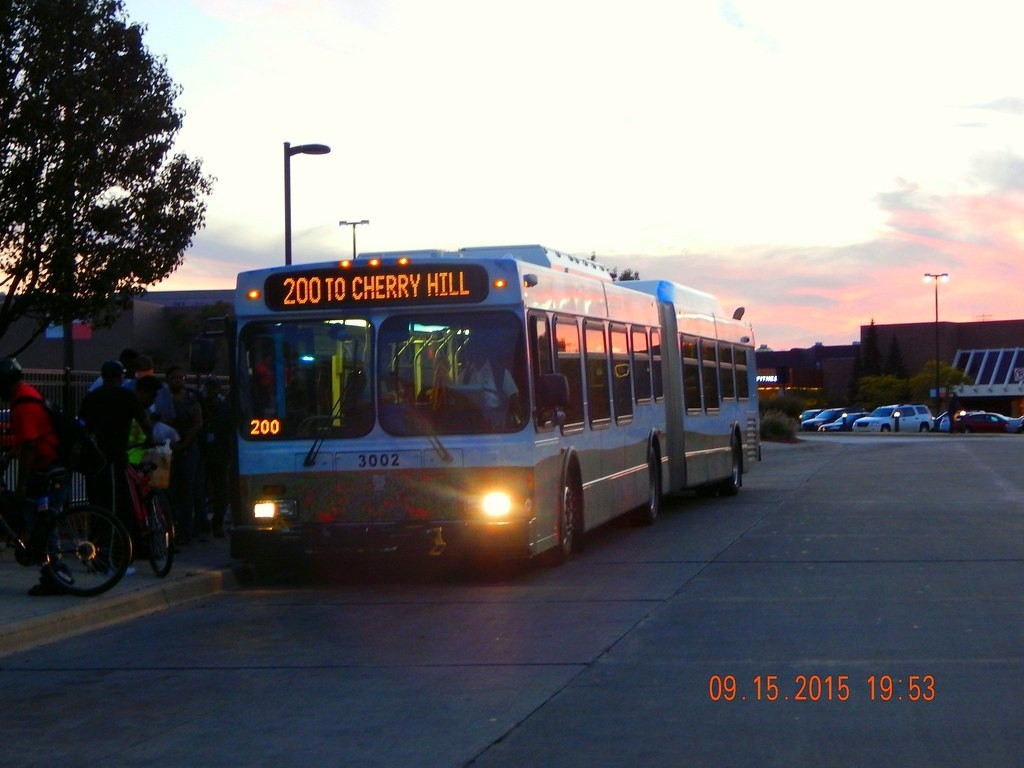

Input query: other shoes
[214,528,226,538]
[199,532,210,542]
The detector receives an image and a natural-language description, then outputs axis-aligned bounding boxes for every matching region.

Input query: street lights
[282,141,332,266]
[923,272,949,417]
[337,218,372,259]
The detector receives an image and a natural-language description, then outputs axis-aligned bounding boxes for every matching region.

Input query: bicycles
[120,440,183,578]
[0,445,133,599]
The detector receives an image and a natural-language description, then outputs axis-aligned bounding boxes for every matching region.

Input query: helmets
[0,357,23,402]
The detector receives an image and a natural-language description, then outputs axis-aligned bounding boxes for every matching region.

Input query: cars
[935,408,1024,434]
[800,407,824,422]
[817,411,873,432]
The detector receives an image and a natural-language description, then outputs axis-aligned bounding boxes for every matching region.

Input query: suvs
[801,406,864,431]
[852,404,936,432]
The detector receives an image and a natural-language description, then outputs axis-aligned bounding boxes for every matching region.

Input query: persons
[948,394,961,432]
[460,339,520,408]
[0,357,73,595]
[80,348,230,575]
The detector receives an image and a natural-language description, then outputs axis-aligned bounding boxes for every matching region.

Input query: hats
[102,360,126,376]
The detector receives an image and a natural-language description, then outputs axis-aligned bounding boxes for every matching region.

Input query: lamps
[960,410,967,417]
[893,411,900,417]
[842,412,848,417]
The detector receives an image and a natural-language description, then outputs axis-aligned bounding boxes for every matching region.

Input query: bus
[188,243,763,571]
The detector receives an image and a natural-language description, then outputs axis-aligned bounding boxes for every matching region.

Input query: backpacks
[11,397,108,479]
